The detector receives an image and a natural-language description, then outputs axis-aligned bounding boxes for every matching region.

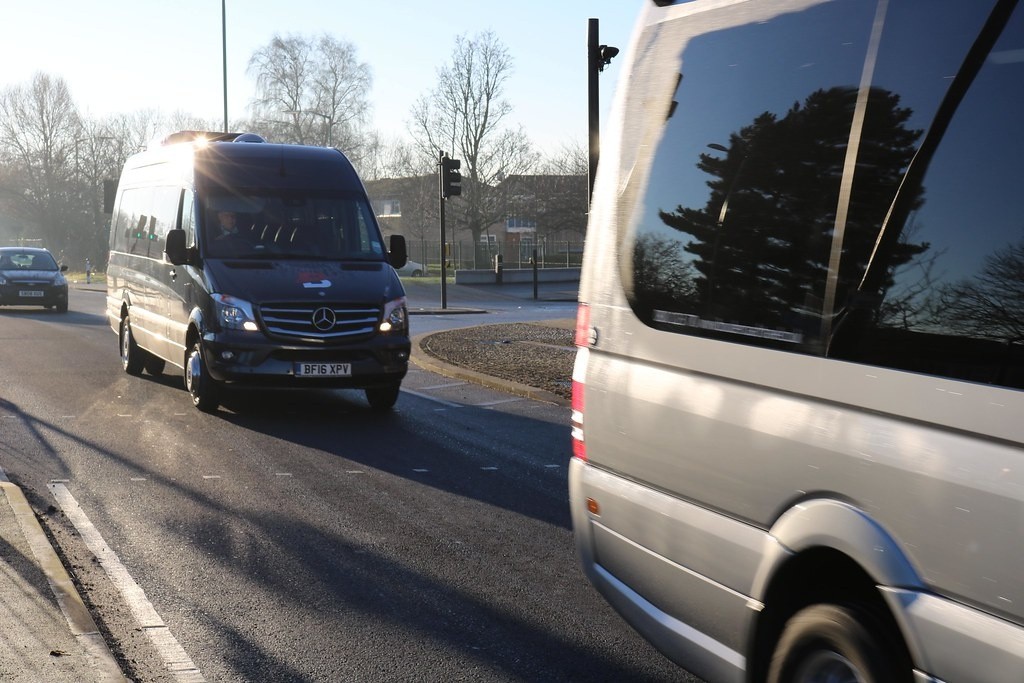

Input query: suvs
[104,131,413,412]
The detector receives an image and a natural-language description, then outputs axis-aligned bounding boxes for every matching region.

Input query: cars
[395,259,429,278]
[0,245,70,312]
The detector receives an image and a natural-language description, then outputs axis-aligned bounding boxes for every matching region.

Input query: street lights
[280,108,333,148]
[72,135,116,201]
[701,142,751,318]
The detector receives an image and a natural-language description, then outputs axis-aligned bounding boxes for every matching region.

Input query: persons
[211,207,247,242]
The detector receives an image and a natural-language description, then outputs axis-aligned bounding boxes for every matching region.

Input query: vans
[563,1,1024,683]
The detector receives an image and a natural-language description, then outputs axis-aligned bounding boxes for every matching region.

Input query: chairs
[251,222,336,248]
[0,255,16,269]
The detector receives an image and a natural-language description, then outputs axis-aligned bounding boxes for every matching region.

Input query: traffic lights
[440,156,462,199]
[124,214,147,239]
[147,216,158,243]
